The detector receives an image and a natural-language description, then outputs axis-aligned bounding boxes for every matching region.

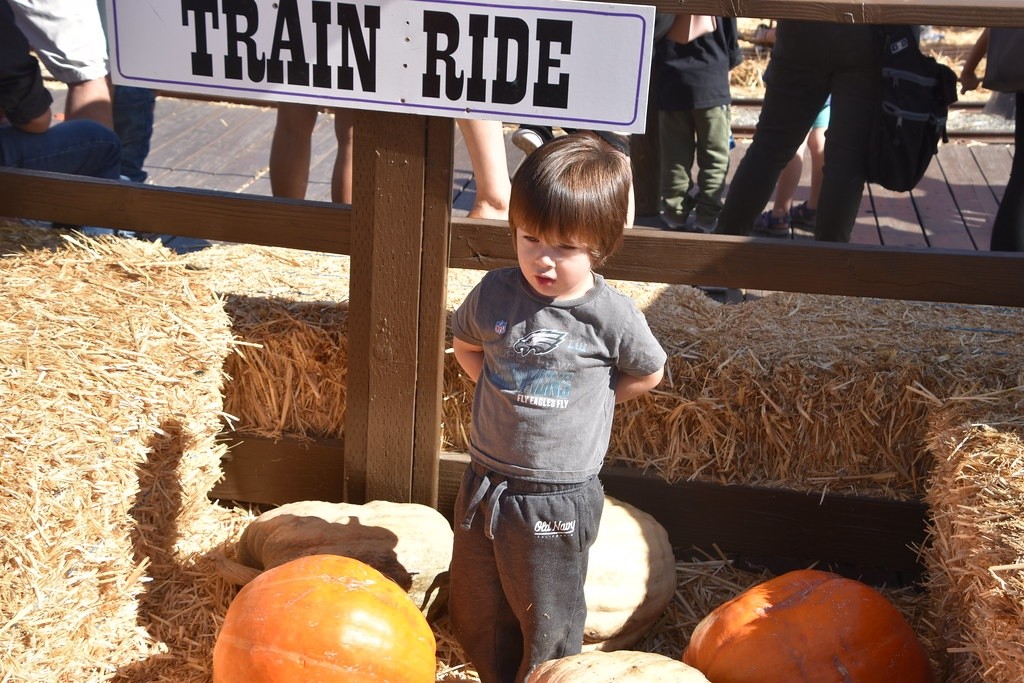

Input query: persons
[455,119,581,220]
[448,131,668,683]
[269,102,352,202]
[0,0,155,241]
[960,27,1024,252]
[627,13,922,291]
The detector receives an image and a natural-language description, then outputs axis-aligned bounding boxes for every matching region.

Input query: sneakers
[511,124,555,157]
[661,216,685,232]
[692,221,718,234]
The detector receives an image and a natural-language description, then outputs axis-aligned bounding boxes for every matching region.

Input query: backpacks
[866,44,960,193]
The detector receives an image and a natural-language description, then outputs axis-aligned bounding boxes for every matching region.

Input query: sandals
[790,200,817,227]
[753,210,790,235]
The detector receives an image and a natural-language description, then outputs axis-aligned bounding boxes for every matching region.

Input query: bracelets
[963,67,974,75]
